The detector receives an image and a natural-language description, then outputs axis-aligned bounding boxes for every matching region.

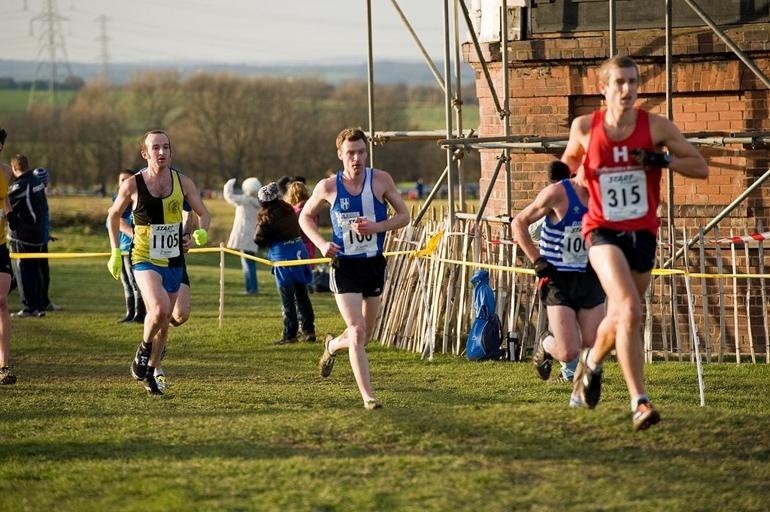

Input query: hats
[33,166,49,184]
[257,182,279,206]
[242,177,261,193]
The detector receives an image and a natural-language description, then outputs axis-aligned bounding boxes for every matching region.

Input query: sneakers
[532,330,660,431]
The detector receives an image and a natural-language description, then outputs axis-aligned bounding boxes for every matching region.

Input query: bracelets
[182,232,192,237]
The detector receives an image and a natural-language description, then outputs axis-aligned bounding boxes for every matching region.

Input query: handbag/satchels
[466,271,504,361]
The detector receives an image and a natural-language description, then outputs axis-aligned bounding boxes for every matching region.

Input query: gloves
[550,161,570,181]
[534,256,554,276]
[107,249,122,279]
[193,229,207,247]
[634,148,662,169]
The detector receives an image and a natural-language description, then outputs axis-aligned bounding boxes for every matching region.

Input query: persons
[223,169,340,346]
[151,202,192,388]
[511,154,608,379]
[560,54,709,433]
[111,168,146,322]
[415,177,426,199]
[298,129,410,409]
[106,130,210,397]
[0,128,62,384]
